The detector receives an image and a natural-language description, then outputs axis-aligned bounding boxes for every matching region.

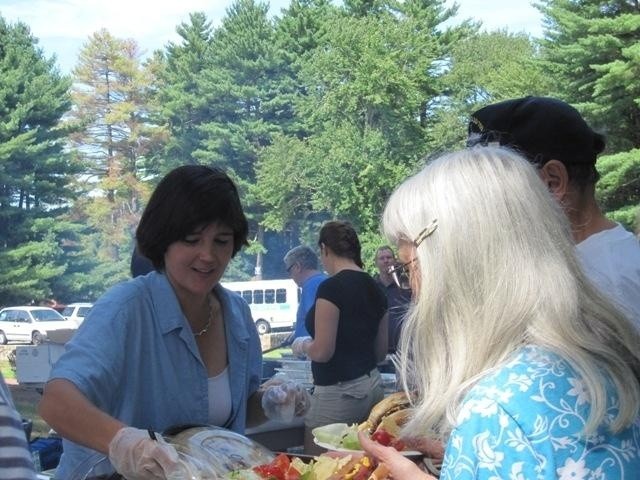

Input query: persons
[357,145,640,480]
[283,220,412,461]
[130,243,157,278]
[466,95,640,329]
[37,165,265,480]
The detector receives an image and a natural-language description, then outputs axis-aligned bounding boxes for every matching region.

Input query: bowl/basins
[163,424,281,480]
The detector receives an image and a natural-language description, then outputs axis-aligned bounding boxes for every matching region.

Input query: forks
[389,352,418,390]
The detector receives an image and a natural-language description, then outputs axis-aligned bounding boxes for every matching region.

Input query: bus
[220,279,302,335]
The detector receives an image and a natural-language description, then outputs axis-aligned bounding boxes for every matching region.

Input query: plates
[311,435,425,457]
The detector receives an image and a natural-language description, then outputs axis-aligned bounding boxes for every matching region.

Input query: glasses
[286,264,293,272]
[387,257,417,290]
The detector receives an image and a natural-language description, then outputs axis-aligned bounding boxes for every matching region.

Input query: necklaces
[192,293,215,337]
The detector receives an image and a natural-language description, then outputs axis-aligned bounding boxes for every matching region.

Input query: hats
[469,96,605,162]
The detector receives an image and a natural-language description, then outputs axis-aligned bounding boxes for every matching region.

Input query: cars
[0,302,93,345]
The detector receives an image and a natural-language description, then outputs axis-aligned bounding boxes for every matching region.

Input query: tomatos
[391,439,406,451]
[371,430,390,446]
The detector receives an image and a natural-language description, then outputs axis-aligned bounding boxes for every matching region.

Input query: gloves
[262,380,310,423]
[292,336,312,357]
[109,427,178,480]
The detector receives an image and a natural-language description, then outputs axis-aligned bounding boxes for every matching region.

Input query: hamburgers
[365,392,416,443]
